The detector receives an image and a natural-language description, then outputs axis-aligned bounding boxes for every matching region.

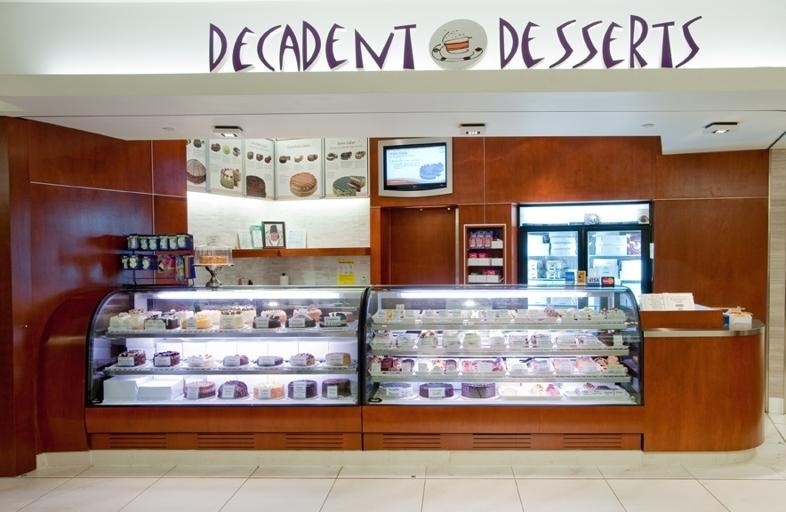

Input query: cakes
[333,176,366,196]
[290,172,317,197]
[187,159,206,184]
[247,176,266,197]
[370,307,627,399]
[107,309,351,398]
[221,169,241,189]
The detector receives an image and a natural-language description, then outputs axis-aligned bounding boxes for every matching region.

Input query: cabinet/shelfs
[463,223,507,284]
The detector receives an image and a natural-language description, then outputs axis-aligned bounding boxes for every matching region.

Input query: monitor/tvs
[378,137,454,198]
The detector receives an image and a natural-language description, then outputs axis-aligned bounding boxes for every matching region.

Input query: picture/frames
[261,221,286,249]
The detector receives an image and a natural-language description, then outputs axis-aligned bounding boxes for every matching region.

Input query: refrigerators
[516,198,658,312]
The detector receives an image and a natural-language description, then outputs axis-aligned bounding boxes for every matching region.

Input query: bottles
[279,273,288,286]
